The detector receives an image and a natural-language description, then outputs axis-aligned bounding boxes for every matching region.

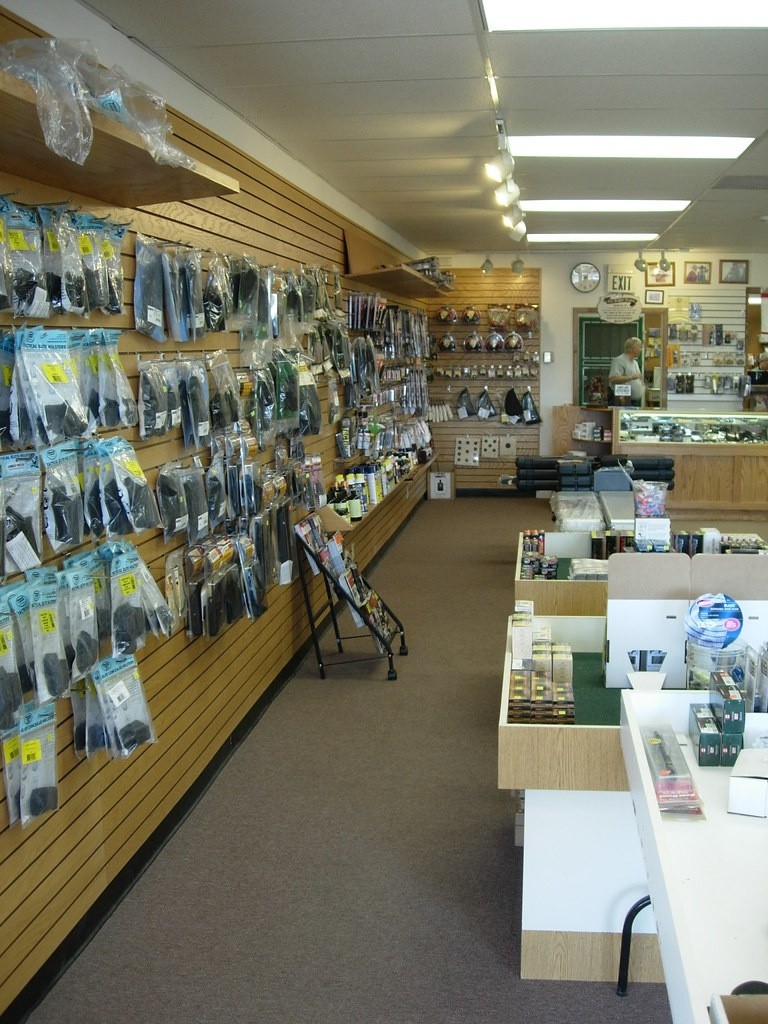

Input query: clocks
[571,262,601,293]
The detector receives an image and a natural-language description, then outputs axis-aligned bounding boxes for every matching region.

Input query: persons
[608,337,645,407]
[747,351,768,384]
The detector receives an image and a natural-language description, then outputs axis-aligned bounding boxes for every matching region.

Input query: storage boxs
[427,472,456,500]
[508,490,768,818]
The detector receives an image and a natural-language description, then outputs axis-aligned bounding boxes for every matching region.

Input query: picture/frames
[684,262,712,284]
[645,290,664,304]
[719,259,749,284]
[645,262,675,287]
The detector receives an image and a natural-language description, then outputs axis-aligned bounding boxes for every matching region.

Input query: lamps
[495,180,520,206]
[660,251,670,272]
[481,254,494,274]
[512,254,524,273]
[502,205,526,229]
[634,251,648,272]
[485,151,515,183]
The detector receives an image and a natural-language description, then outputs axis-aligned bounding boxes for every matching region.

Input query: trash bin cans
[594,466,632,491]
